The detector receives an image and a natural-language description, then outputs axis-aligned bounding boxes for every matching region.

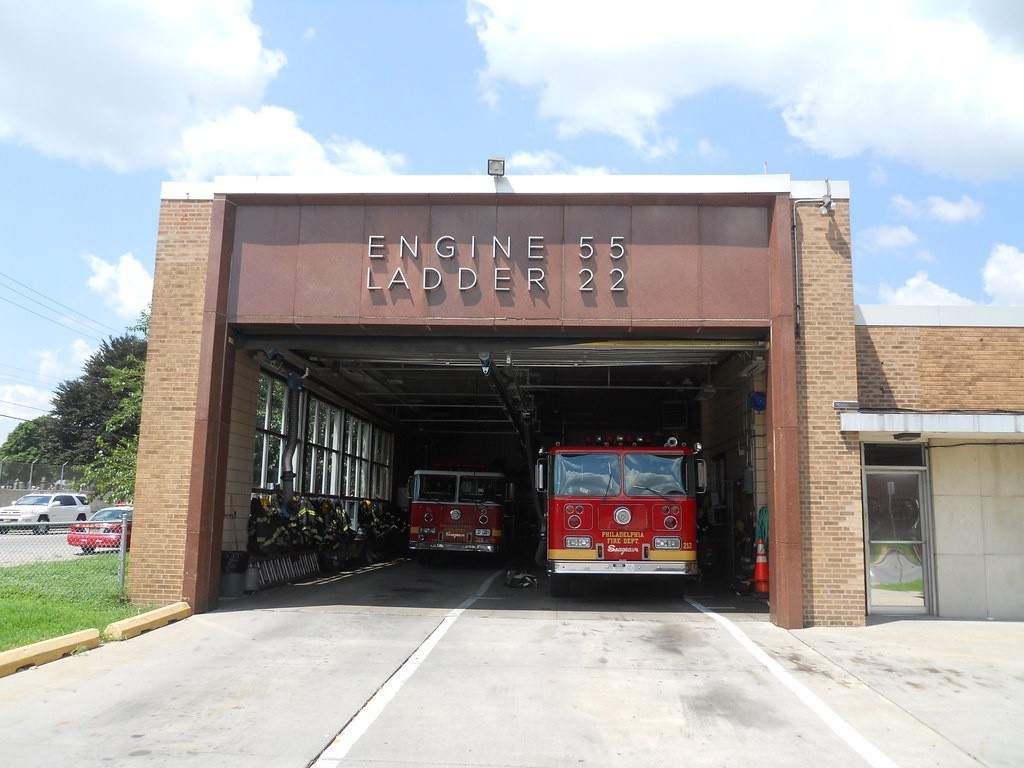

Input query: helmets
[286,500,300,515]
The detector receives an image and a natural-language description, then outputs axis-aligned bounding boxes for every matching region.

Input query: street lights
[59,460,70,492]
[28,459,39,489]
[0,455,9,478]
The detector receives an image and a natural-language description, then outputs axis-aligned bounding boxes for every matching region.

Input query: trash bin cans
[220,550,249,597]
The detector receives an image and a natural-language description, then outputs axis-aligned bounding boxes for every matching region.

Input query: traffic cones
[753,538,769,599]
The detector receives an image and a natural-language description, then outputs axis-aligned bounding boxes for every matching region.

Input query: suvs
[0,491,92,535]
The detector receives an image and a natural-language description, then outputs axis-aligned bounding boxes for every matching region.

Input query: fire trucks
[534,432,709,599]
[406,464,516,566]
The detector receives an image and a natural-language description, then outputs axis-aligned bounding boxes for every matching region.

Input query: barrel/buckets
[244,567,260,591]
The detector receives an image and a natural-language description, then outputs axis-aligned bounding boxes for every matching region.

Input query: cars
[66,503,133,553]
